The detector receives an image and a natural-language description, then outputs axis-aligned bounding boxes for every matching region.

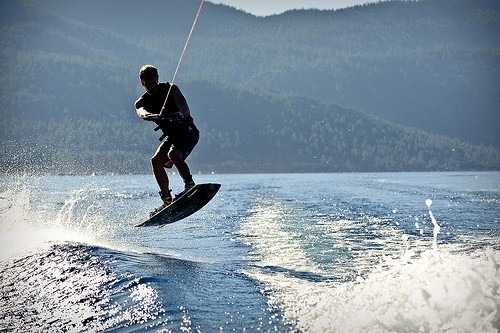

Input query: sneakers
[178,181,195,196]
[157,189,173,211]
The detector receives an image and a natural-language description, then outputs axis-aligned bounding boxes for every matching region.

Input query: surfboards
[136,183,221,228]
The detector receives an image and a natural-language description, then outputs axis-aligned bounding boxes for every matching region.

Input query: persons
[134,65,199,211]
